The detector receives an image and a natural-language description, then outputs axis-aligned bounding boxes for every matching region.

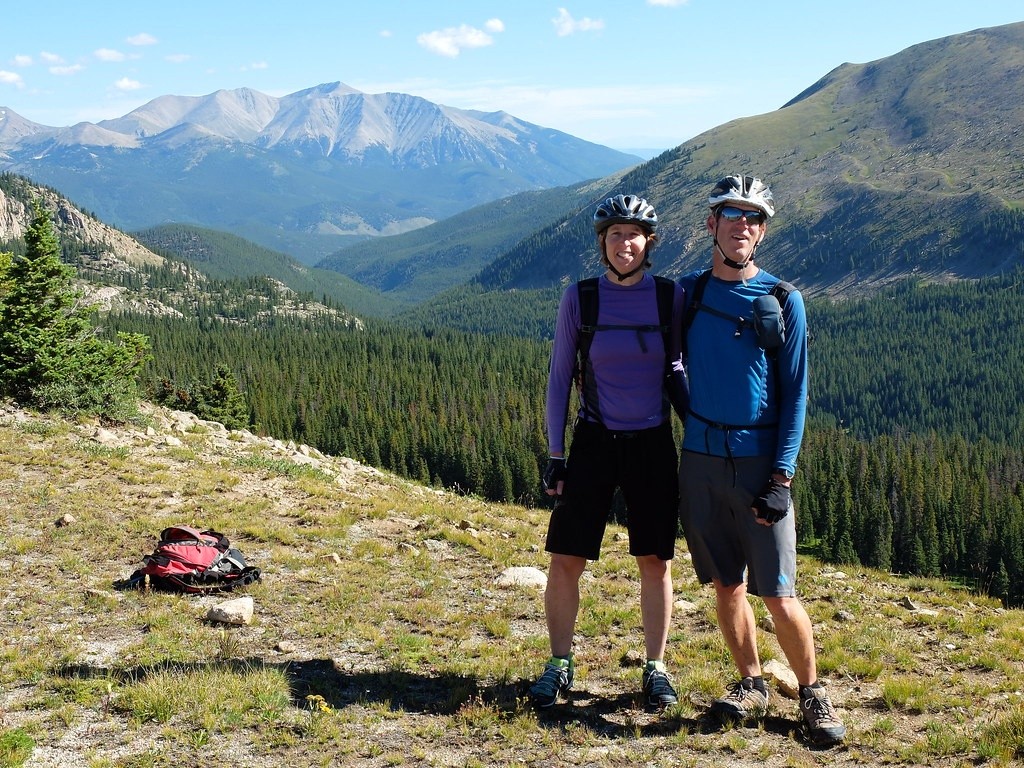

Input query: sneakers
[642,659,678,705]
[529,651,575,707]
[799,684,846,742]
[712,677,768,720]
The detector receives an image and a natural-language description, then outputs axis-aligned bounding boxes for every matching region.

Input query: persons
[530,195,690,707]
[575,174,847,745]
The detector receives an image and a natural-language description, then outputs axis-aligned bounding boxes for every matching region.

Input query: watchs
[771,467,794,479]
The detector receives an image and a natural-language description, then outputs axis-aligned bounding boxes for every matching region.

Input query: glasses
[716,206,765,226]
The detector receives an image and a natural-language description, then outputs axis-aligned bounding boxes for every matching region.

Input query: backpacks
[113,525,261,595]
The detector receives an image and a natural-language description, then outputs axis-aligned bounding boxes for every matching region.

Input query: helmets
[708,172,775,219]
[594,194,658,233]
